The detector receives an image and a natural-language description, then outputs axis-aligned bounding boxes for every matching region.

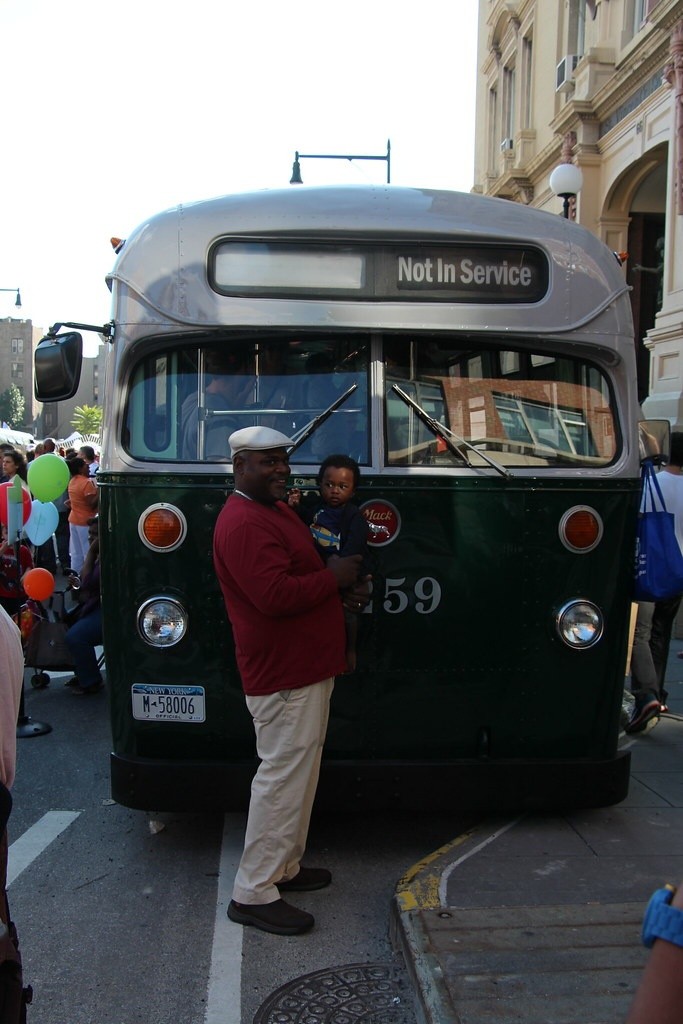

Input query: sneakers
[659,697,668,712]
[274,867,332,891]
[227,899,314,936]
[625,695,661,734]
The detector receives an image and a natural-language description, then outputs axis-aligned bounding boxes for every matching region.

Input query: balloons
[23,567,56,601]
[26,453,71,504]
[0,482,32,527]
[24,499,60,548]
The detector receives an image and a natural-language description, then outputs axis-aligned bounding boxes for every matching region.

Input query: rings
[357,602,361,607]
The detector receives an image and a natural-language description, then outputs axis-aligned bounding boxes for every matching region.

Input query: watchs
[639,884,683,949]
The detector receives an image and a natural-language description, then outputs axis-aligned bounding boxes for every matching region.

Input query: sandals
[66,676,105,697]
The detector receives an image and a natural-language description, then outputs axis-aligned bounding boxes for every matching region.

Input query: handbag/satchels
[24,608,73,669]
[634,461,683,603]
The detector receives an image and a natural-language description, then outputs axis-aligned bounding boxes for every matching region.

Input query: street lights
[289,138,391,186]
[550,163,585,221]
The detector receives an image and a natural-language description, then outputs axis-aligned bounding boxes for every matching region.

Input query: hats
[227,425,296,458]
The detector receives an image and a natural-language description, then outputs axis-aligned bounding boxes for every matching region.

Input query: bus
[31,184,674,816]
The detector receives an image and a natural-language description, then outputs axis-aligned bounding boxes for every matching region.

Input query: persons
[211,425,375,934]
[285,454,371,674]
[0,603,34,1024]
[621,432,683,735]
[0,438,108,693]
[626,882,683,1024]
[170,345,378,467]
